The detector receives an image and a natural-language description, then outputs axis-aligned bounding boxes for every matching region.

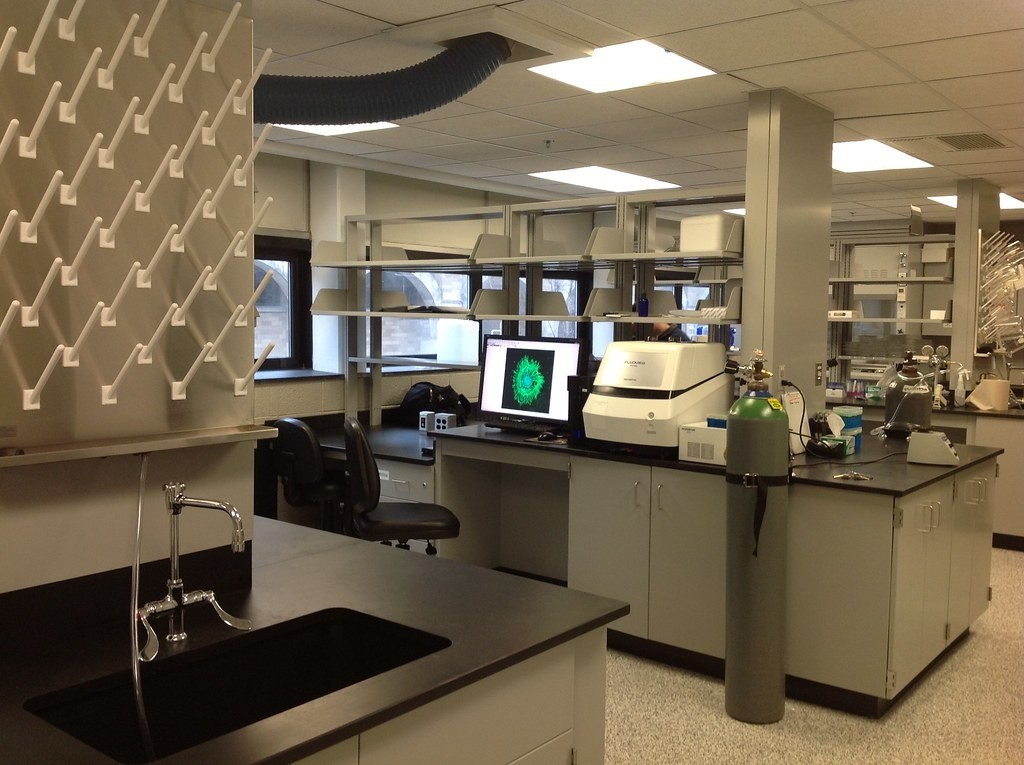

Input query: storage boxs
[679,212,740,251]
[679,422,728,465]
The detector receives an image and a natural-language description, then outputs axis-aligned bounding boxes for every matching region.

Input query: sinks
[21,606,454,765]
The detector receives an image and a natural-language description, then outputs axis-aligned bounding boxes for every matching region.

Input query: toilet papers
[965,379,1011,411]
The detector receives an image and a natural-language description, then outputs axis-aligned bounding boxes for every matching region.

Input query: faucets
[137,480,254,664]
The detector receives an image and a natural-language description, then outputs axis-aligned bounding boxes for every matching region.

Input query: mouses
[538,432,557,441]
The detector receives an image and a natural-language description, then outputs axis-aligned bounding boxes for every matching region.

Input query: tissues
[820,413,855,457]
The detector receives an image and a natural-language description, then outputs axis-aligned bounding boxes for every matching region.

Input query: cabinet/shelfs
[310,187,746,327]
[827,237,956,361]
[275,403,1024,765]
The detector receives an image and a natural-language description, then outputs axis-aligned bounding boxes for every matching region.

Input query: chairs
[270,417,349,518]
[342,418,460,556]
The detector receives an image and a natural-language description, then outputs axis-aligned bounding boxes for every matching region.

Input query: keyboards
[484,420,561,433]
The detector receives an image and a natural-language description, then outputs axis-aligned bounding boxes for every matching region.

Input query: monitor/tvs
[478,334,585,426]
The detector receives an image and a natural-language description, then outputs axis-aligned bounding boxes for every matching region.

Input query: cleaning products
[954,368,972,408]
